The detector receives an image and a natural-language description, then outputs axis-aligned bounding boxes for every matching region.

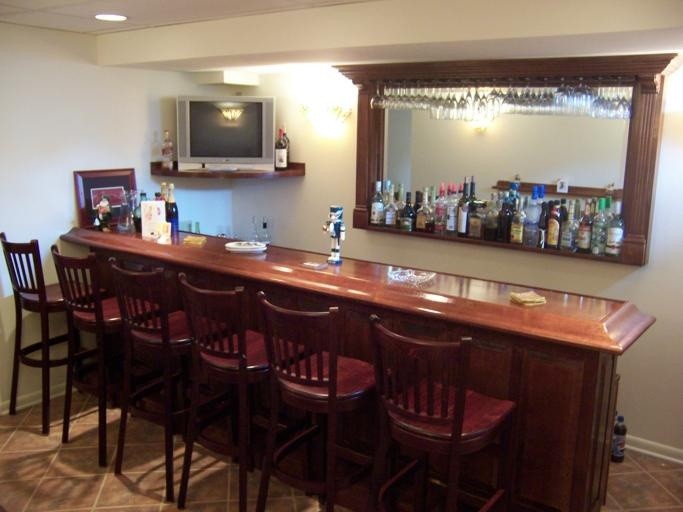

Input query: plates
[224,241,268,254]
[388,267,438,286]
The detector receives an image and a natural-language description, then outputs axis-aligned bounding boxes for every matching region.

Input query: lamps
[221,108,243,120]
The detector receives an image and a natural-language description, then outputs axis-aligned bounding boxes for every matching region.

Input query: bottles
[369,178,623,256]
[610,416,626,462]
[276,127,291,169]
[117,183,179,237]
[161,129,174,171]
[248,215,271,245]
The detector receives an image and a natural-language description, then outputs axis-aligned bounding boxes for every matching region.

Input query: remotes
[208,166,237,172]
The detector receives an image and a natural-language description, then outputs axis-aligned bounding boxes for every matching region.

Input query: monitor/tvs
[177,95,275,172]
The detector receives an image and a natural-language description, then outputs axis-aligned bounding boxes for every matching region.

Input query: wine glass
[369,78,632,123]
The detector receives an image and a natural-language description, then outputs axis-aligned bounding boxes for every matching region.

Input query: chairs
[0,232,100,434]
[177,273,309,511]
[107,256,239,504]
[368,314,518,511]
[255,291,377,512]
[51,244,162,466]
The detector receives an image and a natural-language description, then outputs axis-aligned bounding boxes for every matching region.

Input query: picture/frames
[73,168,136,229]
